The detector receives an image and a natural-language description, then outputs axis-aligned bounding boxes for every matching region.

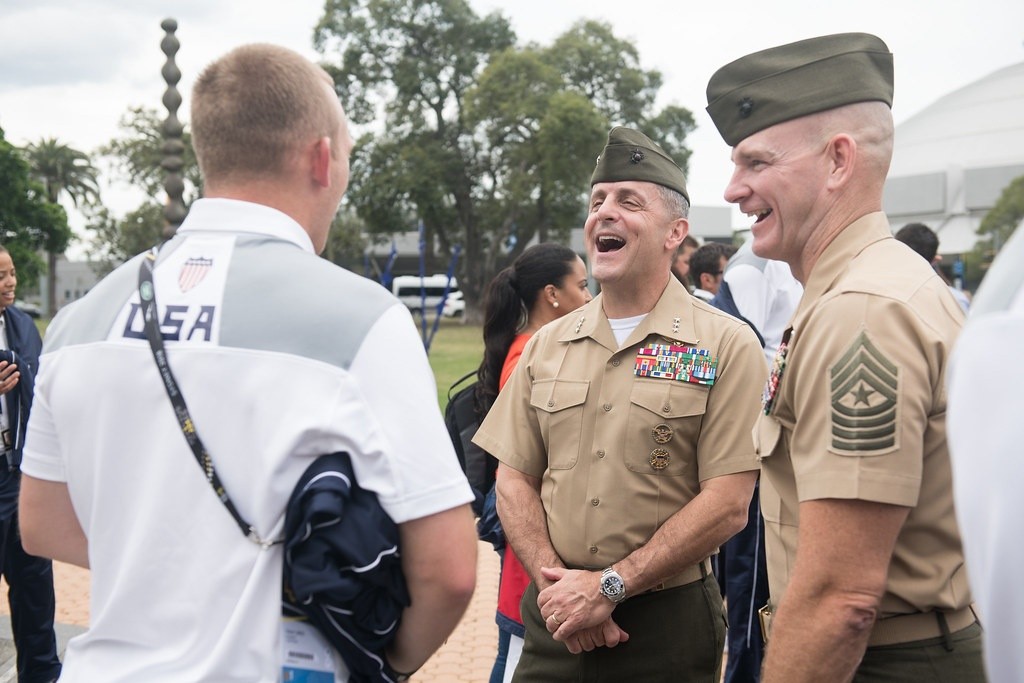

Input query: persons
[0,245,63,683]
[472,224,1024,683]
[704,31,992,683]
[470,127,769,683]
[14,41,478,683]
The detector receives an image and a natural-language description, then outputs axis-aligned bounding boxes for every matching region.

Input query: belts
[758,602,979,646]
[0,448,13,475]
[650,555,712,592]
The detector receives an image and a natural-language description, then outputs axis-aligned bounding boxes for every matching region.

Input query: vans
[393,274,459,317]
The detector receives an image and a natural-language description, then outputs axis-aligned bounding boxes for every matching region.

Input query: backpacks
[444,369,499,518]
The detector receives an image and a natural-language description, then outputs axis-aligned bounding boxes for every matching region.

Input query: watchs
[599,566,628,604]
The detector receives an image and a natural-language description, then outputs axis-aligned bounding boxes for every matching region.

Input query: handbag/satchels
[473,484,508,561]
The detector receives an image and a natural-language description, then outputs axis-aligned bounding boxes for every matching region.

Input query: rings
[552,613,561,624]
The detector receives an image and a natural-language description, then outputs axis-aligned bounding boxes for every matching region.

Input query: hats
[706,32,894,146]
[591,127,690,207]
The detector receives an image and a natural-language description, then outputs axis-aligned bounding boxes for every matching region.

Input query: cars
[441,290,466,318]
[12,295,41,321]
